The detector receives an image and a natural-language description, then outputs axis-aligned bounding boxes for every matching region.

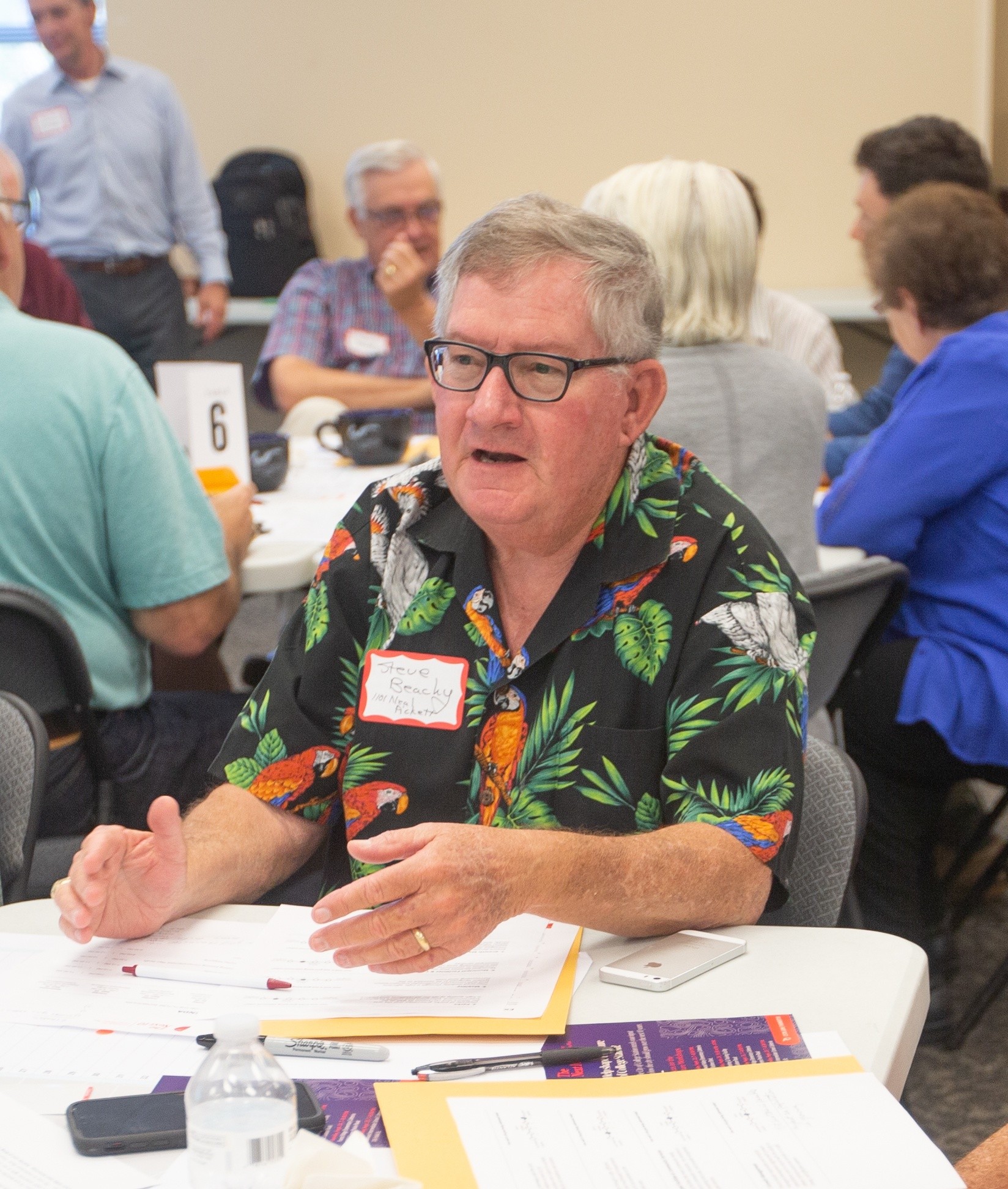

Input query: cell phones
[66,1081,328,1157]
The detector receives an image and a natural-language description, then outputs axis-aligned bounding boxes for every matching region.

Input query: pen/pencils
[410,1046,617,1081]
[195,1034,389,1062]
[121,964,291,989]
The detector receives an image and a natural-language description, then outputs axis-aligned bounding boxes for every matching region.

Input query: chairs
[0,556,1006,1052]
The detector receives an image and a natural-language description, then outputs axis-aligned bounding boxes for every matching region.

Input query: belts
[39,708,108,739]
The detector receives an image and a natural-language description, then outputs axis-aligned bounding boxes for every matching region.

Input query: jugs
[316,409,413,466]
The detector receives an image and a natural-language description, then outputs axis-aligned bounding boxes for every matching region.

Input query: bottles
[185,1017,297,1189]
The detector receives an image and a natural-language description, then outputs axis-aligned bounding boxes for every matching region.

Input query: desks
[221,436,411,693]
[0,895,933,1189]
[183,296,897,349]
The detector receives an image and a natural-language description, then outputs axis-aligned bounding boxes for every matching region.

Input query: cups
[248,433,288,492]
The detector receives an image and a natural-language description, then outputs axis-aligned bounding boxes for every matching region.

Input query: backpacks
[213,152,318,296]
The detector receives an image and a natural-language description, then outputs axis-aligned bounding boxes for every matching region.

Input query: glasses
[370,202,440,228]
[0,193,31,229]
[873,297,887,316]
[423,336,635,402]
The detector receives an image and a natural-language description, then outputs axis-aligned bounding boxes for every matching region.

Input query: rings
[413,927,430,951]
[384,263,397,276]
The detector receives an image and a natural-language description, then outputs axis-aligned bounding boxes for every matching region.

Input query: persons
[50,194,817,973]
[0,0,1008,1046]
